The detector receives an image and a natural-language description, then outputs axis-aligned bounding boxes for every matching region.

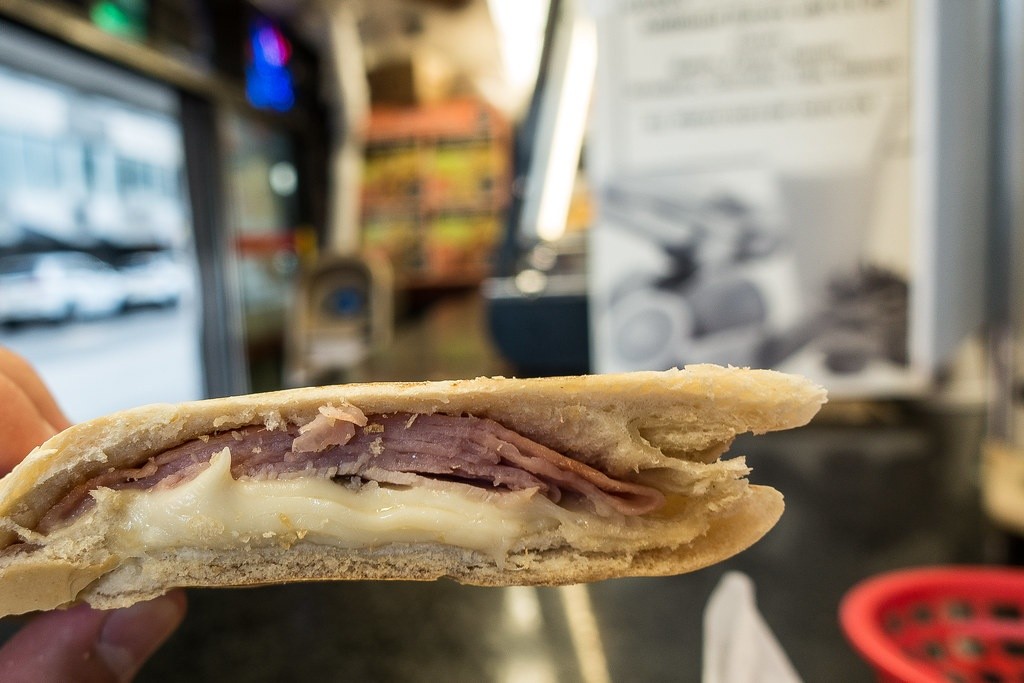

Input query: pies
[0,364,827,621]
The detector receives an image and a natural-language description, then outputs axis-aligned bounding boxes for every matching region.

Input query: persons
[0,351,188,683]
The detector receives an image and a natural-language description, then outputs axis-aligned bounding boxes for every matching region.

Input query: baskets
[839,567,1024,683]
